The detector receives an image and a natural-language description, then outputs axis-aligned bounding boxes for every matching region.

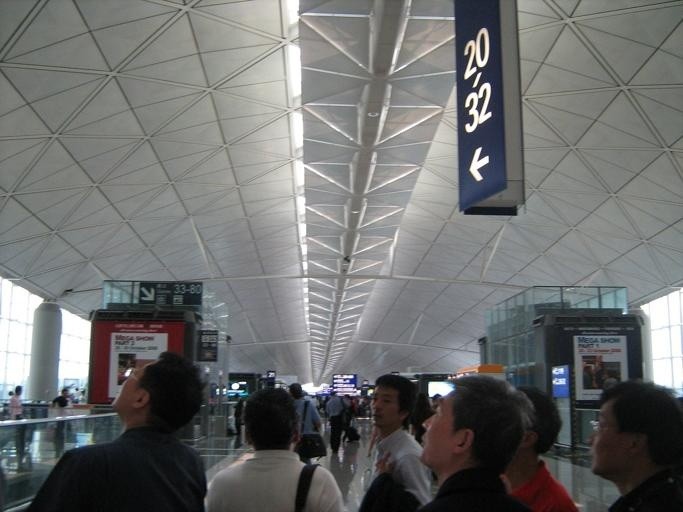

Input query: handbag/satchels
[295,433,326,458]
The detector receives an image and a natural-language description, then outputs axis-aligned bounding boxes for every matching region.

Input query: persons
[52,386,85,420]
[25,352,207,512]
[9,386,23,420]
[207,375,683,511]
